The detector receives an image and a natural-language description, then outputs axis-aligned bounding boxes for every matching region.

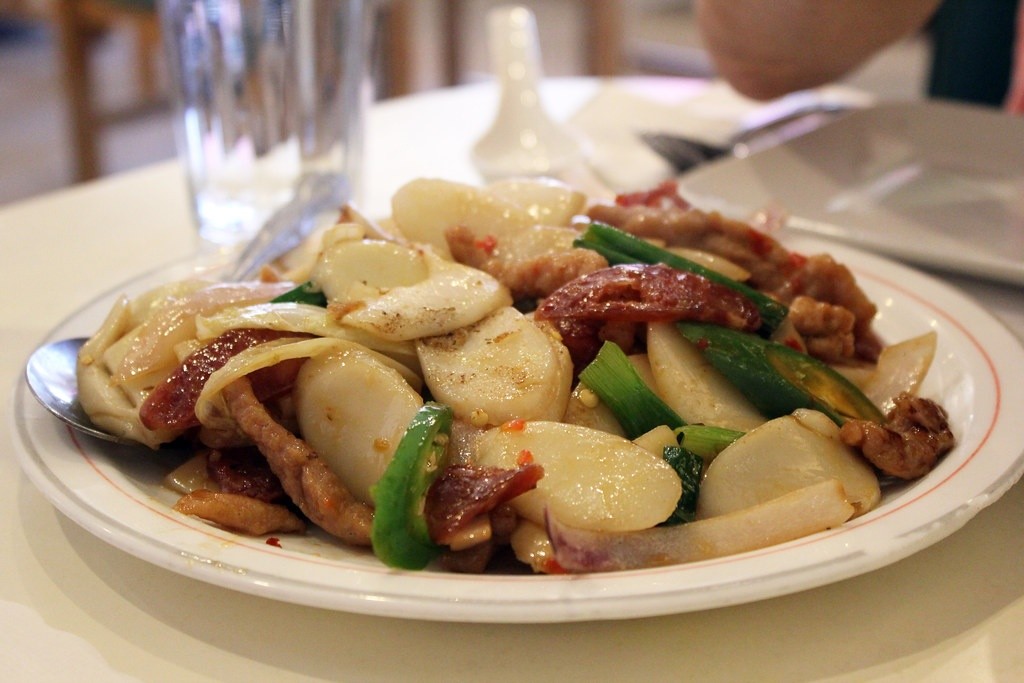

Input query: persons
[691,0,1024,118]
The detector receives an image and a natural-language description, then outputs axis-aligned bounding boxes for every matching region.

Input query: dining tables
[2,73,1024,683]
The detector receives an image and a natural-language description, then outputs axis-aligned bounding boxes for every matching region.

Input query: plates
[676,93,1024,286]
[6,227,1024,628]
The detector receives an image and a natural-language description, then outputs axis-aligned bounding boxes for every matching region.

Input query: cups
[158,0,378,243]
